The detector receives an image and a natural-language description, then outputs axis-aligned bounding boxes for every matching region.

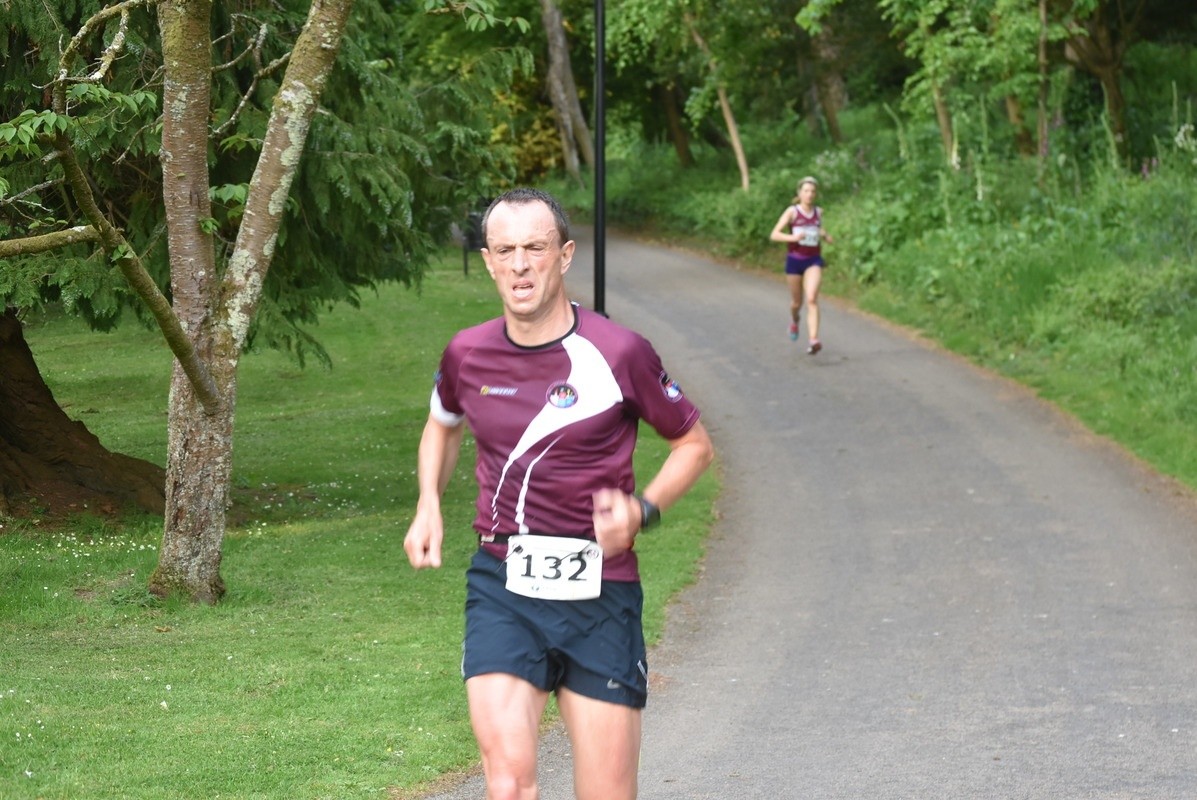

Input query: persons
[770,177,833,354]
[402,186,715,800]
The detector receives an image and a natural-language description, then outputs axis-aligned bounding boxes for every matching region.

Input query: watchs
[636,495,661,534]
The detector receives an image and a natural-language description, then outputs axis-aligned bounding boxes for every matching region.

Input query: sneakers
[789,322,799,341]
[806,340,821,354]
